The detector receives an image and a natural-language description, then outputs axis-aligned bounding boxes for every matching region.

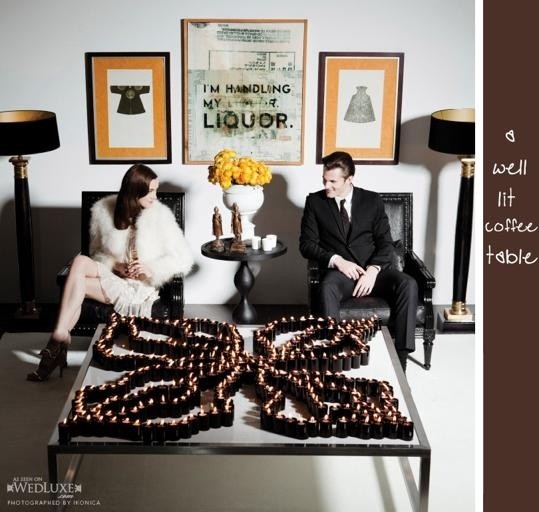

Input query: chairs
[302,192,434,369]
[56,192,189,378]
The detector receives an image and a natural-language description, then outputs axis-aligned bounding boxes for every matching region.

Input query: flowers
[208,150,273,187]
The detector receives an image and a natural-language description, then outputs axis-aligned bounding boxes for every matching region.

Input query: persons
[299,151,417,379]
[210,206,224,253]
[26,164,195,382]
[229,203,246,255]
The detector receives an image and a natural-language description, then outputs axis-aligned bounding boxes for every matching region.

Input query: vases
[222,183,265,248]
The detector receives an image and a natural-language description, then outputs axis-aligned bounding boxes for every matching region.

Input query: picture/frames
[84,51,173,165]
[316,51,404,166]
[181,18,307,166]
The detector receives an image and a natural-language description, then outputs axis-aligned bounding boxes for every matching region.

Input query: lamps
[0,109,62,332]
[426,107,476,334]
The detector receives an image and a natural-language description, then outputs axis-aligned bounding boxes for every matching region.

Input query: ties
[340,199,350,238]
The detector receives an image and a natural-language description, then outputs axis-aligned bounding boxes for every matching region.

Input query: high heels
[36,331,71,370]
[27,356,63,382]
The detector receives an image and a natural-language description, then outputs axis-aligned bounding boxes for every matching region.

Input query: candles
[58,312,414,442]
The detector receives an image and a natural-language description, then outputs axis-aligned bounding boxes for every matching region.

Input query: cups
[262,239,272,251]
[267,235,277,247]
[130,250,139,263]
[251,236,261,249]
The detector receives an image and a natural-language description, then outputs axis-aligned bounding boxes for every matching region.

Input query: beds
[45,325,432,511]
